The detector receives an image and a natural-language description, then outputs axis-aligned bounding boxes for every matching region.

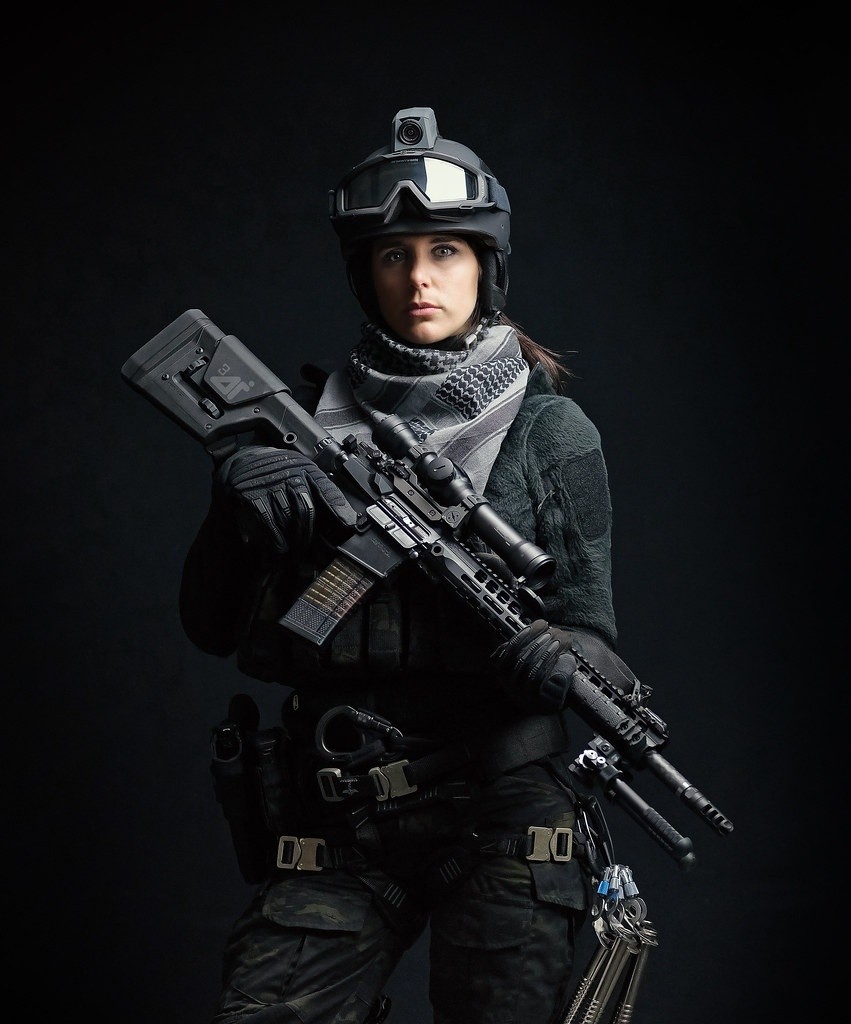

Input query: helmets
[424,123,426,126]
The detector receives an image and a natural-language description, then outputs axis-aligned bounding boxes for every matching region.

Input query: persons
[179,107,627,1024]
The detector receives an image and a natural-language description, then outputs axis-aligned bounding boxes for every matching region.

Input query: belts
[284,713,577,806]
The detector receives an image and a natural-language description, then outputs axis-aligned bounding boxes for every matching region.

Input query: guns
[124,305,737,866]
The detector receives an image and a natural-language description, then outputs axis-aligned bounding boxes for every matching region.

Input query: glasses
[326,150,511,234]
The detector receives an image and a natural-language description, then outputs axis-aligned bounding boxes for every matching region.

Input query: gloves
[489,618,577,713]
[213,443,358,553]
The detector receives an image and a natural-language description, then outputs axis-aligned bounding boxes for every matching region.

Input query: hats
[327,106,516,262]
